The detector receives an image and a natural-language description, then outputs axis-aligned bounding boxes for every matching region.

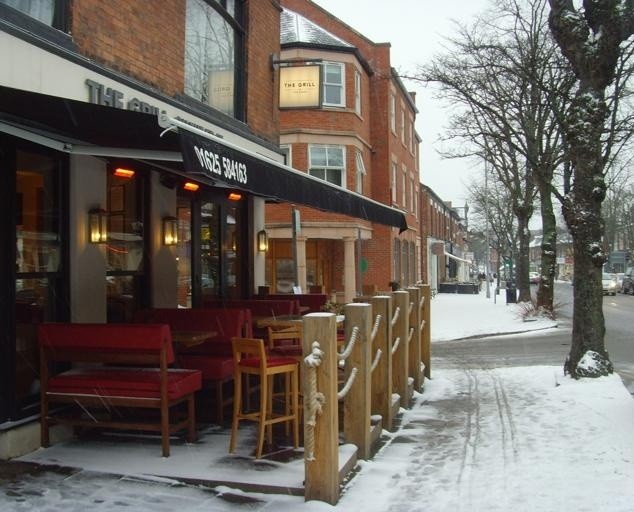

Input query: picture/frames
[110,183,124,242]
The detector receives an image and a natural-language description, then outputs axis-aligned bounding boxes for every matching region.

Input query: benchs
[35,293,327,458]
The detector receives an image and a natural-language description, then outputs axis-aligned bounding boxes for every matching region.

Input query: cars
[529,271,541,285]
[602,266,634,296]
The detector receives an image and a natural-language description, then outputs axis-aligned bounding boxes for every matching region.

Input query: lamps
[112,166,137,181]
[162,216,178,247]
[256,229,268,251]
[88,209,110,244]
